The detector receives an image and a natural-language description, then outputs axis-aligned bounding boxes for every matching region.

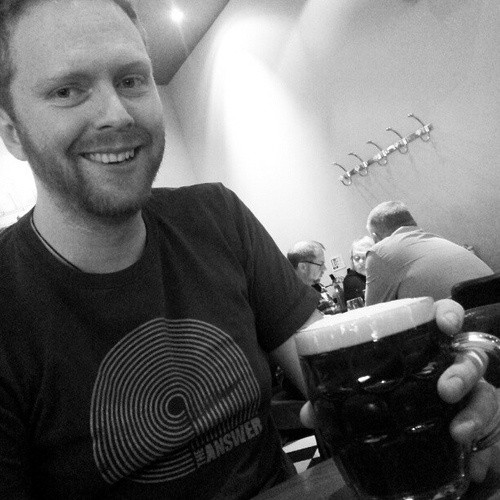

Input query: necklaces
[32,206,84,274]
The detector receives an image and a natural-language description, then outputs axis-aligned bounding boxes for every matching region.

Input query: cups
[347,297,365,311]
[295,296,500,500]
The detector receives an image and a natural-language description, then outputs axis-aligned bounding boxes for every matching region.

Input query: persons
[0,0,500,500]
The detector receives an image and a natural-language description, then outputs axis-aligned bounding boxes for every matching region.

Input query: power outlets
[329,256,345,272]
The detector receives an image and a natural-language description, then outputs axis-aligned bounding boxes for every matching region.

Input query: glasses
[296,260,325,270]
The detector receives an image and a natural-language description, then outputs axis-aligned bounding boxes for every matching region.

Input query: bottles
[329,275,346,311]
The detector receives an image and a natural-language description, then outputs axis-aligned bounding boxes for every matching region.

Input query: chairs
[452,271,500,313]
